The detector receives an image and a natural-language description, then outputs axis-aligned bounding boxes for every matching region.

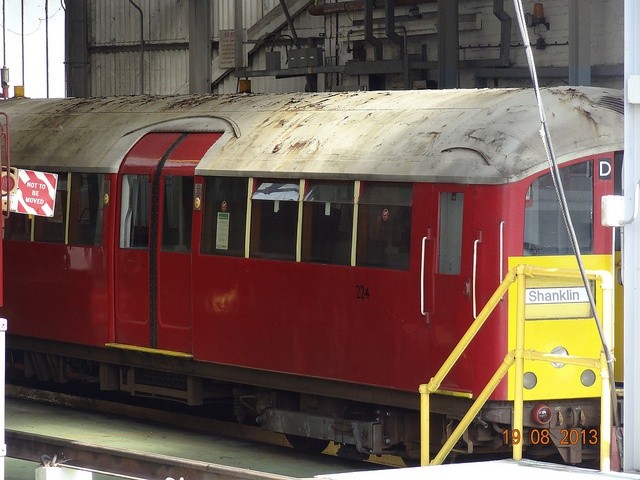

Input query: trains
[0,66,625,472]
